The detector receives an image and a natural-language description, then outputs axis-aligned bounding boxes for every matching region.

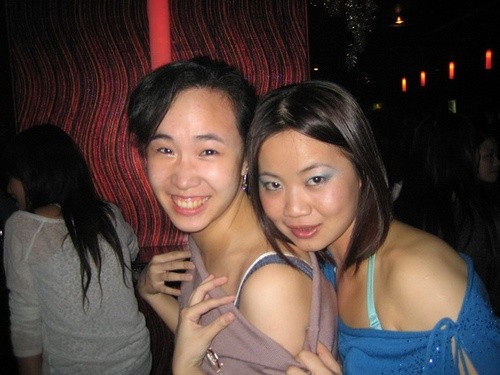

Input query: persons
[377,95,500,317]
[125,54,341,375]
[3,122,153,375]
[236,78,500,375]
